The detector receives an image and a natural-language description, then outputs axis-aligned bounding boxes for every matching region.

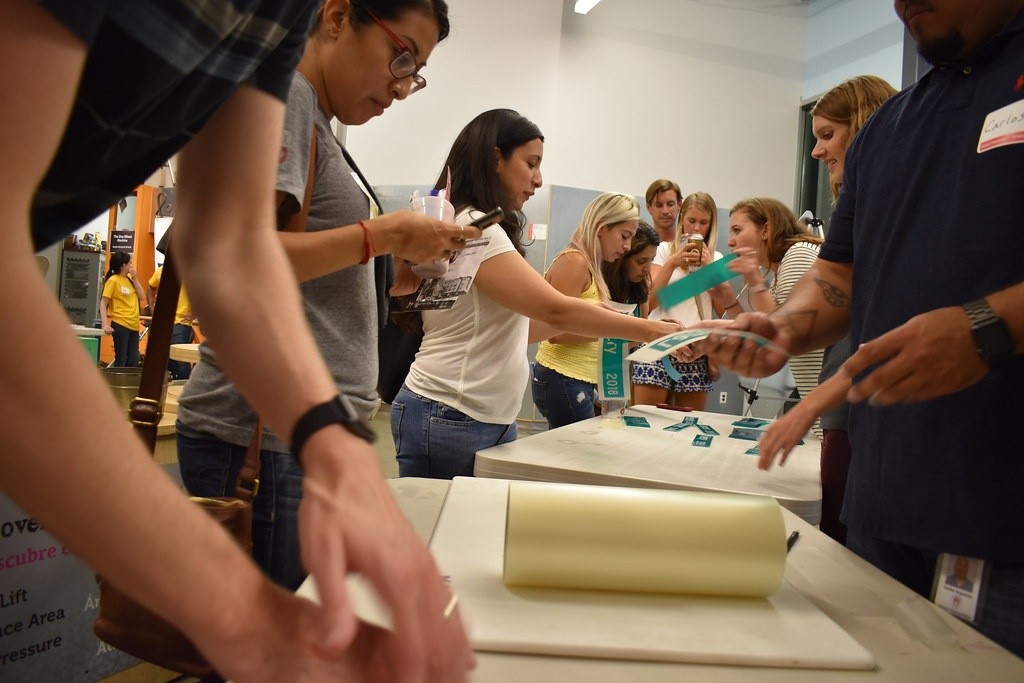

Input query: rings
[441,250,451,262]
[442,596,459,618]
[686,258,688,262]
[455,226,464,241]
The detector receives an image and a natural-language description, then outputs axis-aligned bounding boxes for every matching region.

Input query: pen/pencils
[787,530,799,552]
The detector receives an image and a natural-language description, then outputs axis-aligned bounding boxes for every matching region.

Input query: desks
[473,404,821,527]
[289,476,1024,683]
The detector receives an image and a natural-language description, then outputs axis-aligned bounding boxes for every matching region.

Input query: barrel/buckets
[99,368,144,418]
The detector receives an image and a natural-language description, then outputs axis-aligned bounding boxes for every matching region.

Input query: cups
[407,196,456,278]
[688,238,703,266]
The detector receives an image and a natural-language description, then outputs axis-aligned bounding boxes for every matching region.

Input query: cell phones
[467,207,504,230]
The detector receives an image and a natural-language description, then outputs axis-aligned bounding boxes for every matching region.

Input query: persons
[174,0,482,596]
[100,250,147,367]
[646,180,683,243]
[531,192,640,430]
[946,555,973,593]
[0,0,478,683]
[146,265,196,380]
[601,221,660,354]
[684,0,1024,662]
[631,193,724,411]
[707,197,826,443]
[390,109,692,480]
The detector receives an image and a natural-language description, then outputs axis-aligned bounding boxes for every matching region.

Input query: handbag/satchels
[93,493,253,679]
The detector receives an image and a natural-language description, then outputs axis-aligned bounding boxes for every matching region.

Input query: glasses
[316,0,427,96]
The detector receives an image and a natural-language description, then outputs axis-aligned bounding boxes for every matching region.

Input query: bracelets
[358,221,376,264]
[724,299,739,309]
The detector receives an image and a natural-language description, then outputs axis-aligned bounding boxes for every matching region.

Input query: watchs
[750,279,770,293]
[961,297,1014,371]
[290,394,380,467]
